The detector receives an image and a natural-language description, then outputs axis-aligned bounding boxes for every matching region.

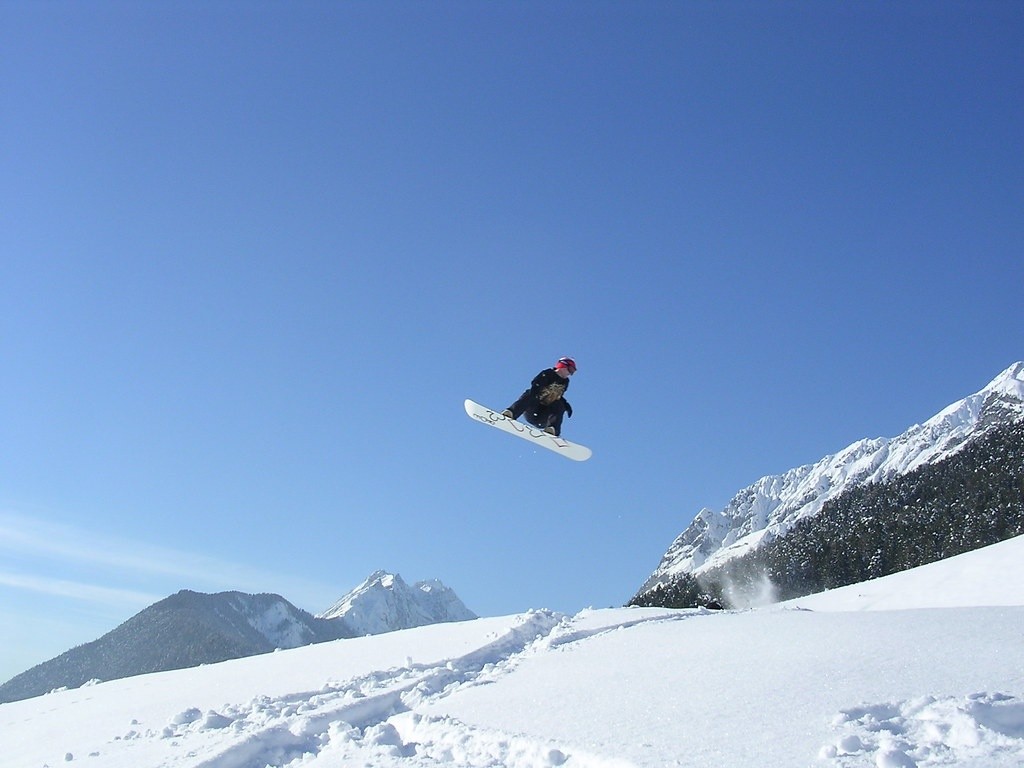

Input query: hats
[556,357,576,368]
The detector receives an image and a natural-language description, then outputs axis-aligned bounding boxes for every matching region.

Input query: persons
[501,357,577,436]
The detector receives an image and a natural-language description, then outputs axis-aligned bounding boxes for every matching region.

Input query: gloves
[566,407,572,418]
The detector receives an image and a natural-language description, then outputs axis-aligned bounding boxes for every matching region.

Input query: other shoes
[501,409,513,418]
[546,427,555,435]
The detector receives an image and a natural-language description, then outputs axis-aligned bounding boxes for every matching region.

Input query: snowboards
[464,398,592,461]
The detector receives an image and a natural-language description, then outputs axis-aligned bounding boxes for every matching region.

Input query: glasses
[566,365,576,374]
